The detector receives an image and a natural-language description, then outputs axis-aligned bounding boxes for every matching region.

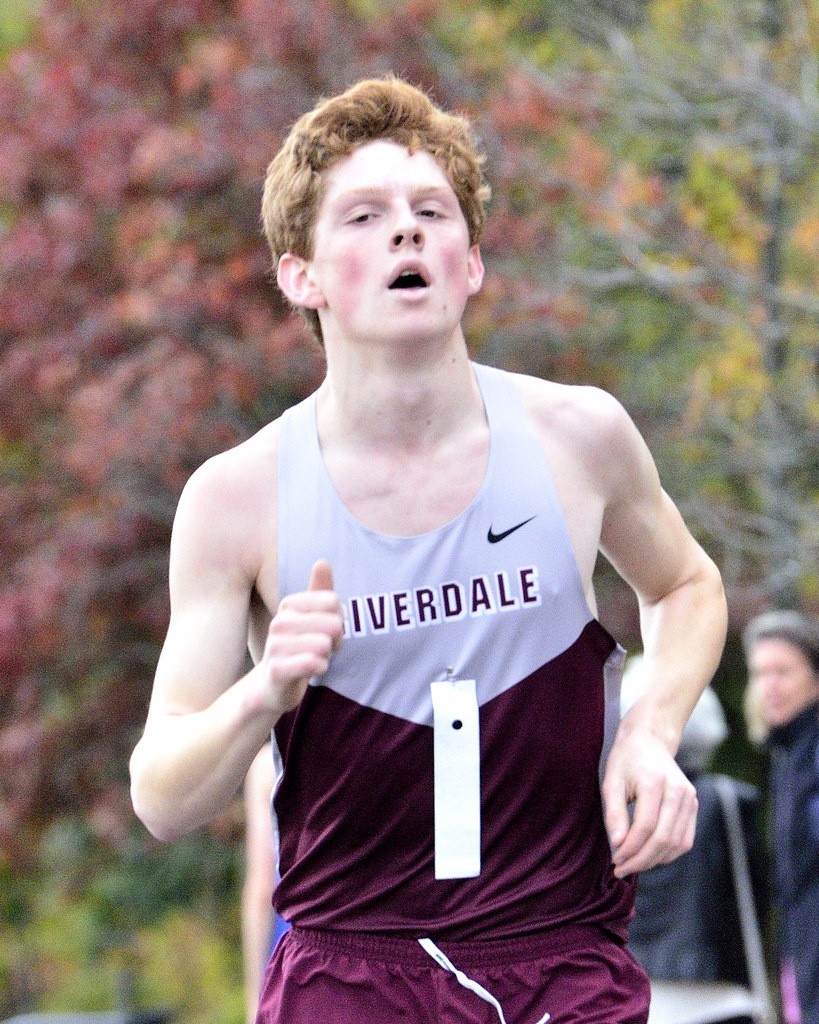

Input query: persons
[617,609,819,1024]
[242,741,293,1024]
[128,78,729,1024]
